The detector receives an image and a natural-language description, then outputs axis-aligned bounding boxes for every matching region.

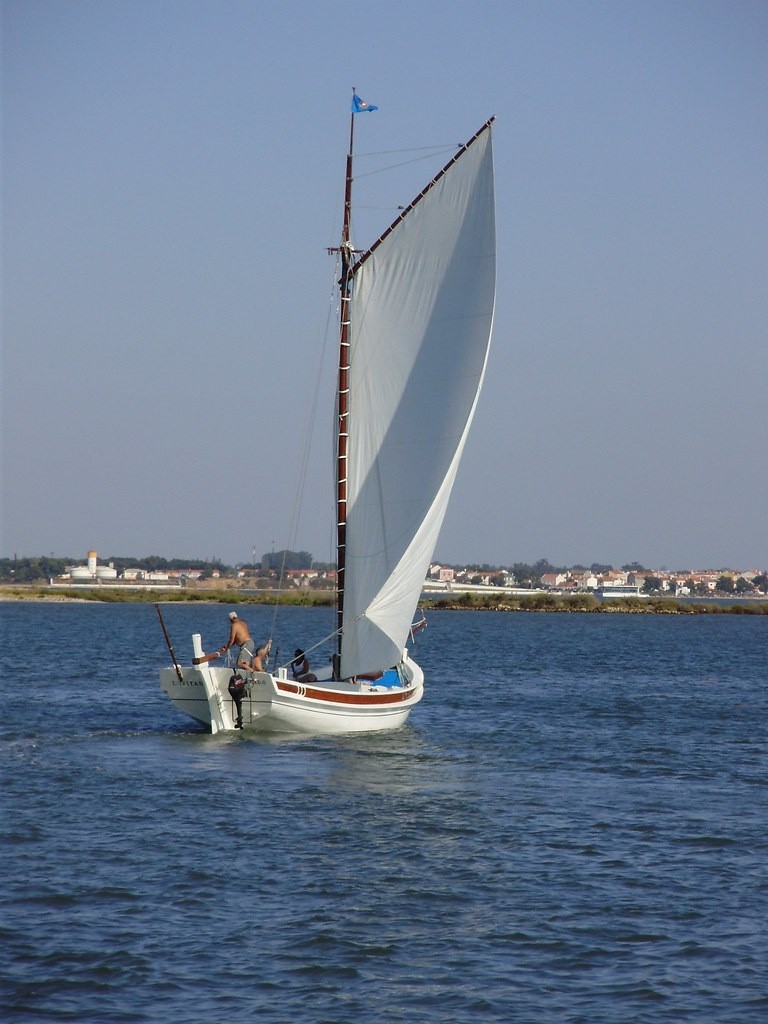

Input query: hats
[229,611,238,619]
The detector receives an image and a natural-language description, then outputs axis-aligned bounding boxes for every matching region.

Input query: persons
[251,638,273,672]
[291,648,317,683]
[220,612,255,672]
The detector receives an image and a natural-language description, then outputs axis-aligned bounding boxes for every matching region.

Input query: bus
[598,586,642,598]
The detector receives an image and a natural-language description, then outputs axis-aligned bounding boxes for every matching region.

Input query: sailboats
[154,79,496,735]
